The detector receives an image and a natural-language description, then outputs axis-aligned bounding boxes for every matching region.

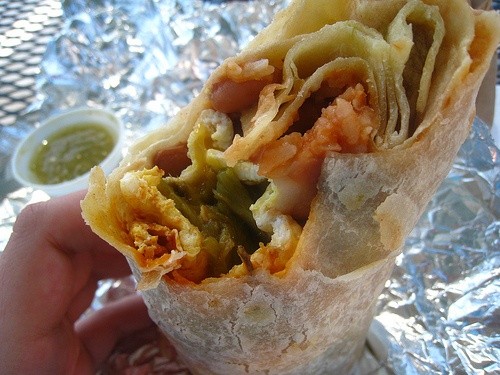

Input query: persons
[0,188,179,374]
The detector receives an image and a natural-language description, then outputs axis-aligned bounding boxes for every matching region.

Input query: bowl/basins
[12,108,125,198]
